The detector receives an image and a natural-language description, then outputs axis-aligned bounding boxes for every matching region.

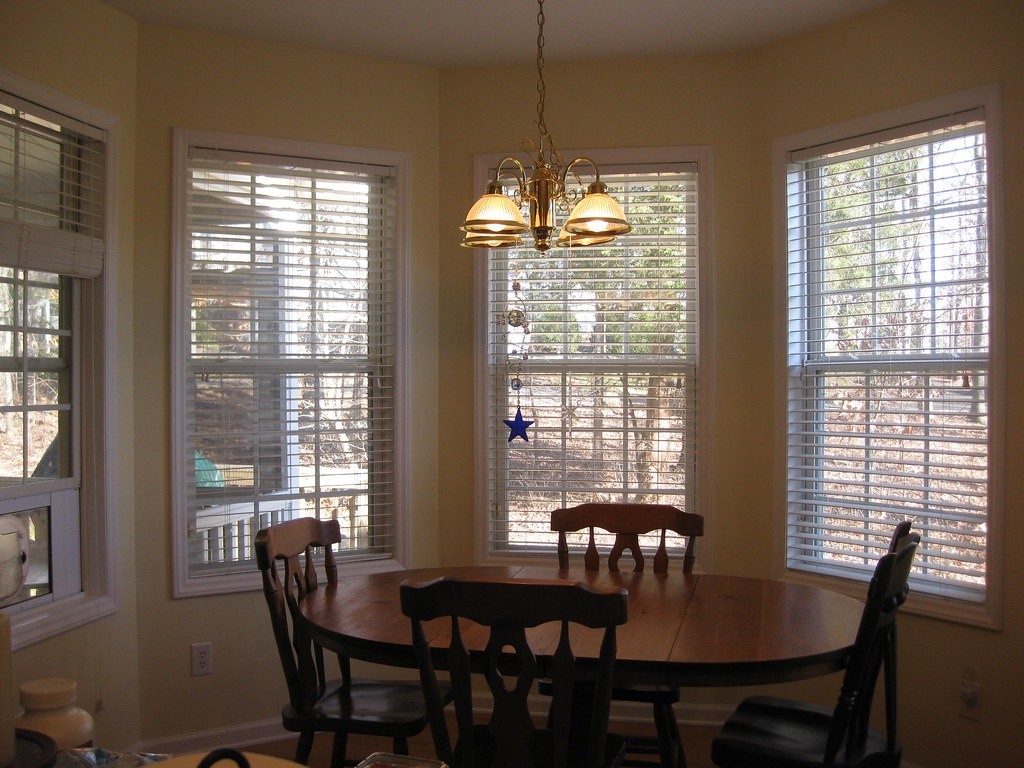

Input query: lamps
[458,0,634,252]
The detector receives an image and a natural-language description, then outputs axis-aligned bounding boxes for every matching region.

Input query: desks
[300,568,866,768]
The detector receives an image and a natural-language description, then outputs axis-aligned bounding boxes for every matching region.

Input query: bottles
[14,676,96,768]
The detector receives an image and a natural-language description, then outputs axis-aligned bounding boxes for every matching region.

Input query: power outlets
[190,641,214,676]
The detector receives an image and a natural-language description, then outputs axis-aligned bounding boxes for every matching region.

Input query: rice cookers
[0,516,29,608]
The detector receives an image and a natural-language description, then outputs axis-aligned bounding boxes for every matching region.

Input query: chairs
[711,518,921,768]
[254,516,454,768]
[399,579,627,768]
[537,503,704,768]
